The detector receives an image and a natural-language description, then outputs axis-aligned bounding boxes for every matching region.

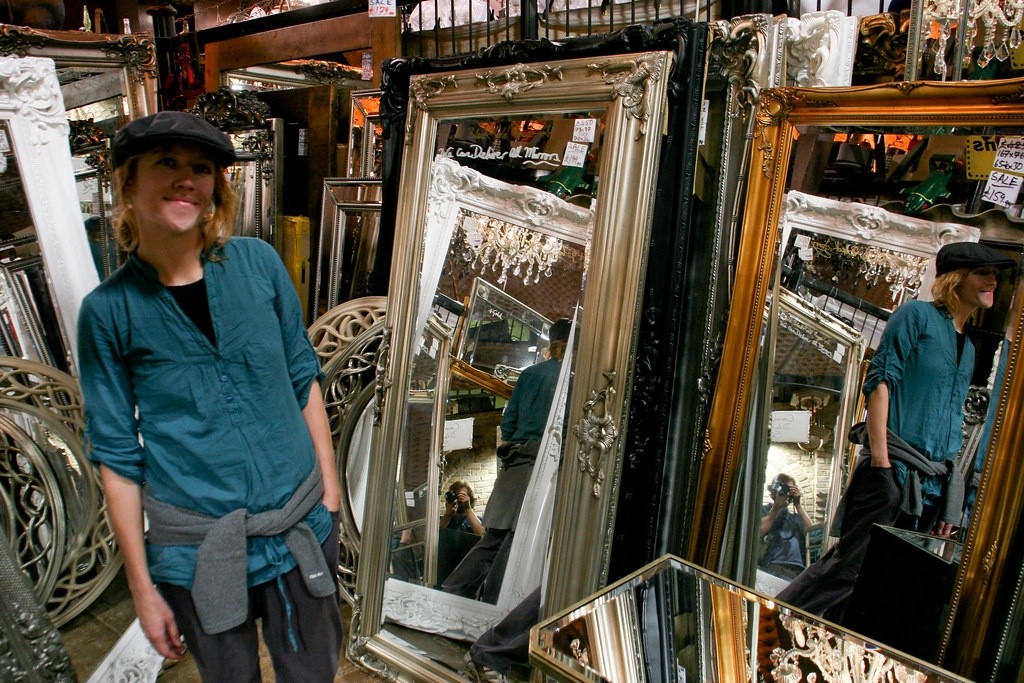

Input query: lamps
[458,210,563,286]
[794,388,835,459]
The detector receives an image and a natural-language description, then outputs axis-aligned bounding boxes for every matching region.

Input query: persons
[757,473,814,582]
[464,586,541,683]
[438,480,484,585]
[438,316,580,606]
[75,108,345,682]
[775,243,1017,618]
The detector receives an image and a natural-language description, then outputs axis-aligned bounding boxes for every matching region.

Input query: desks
[527,554,978,683]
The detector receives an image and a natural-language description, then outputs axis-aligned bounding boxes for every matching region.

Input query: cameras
[445,492,458,504]
[774,482,791,497]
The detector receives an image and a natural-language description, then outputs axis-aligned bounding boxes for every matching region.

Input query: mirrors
[60,72,284,282]
[345,14,708,683]
[0,56,151,551]
[723,190,980,603]
[682,74,1024,683]
[209,17,397,326]
[375,156,596,643]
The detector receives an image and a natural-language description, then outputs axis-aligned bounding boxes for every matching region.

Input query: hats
[110,111,235,167]
[936,241,1018,277]
[550,318,580,349]
